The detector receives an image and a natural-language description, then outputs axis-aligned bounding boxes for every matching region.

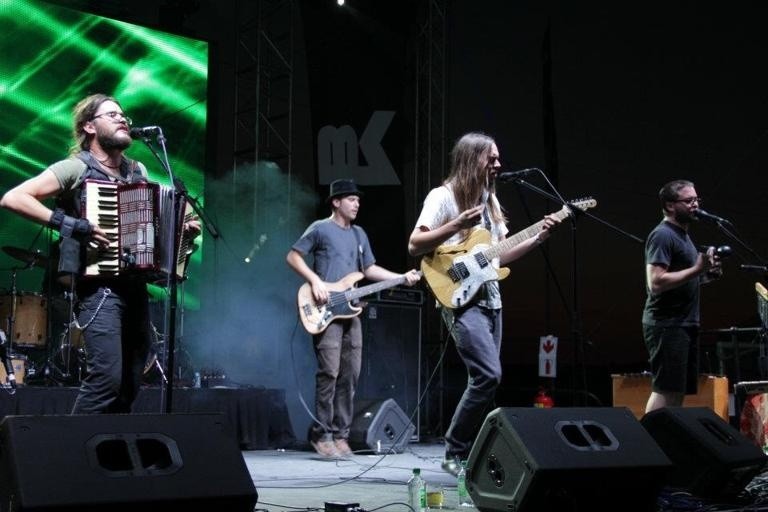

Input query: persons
[1,92,203,414]
[642,180,723,413]
[286,178,422,459]
[406,133,564,464]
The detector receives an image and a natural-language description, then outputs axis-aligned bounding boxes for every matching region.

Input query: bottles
[407,468,426,511]
[457,459,473,510]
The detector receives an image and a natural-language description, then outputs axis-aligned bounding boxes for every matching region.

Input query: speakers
[640,405,768,511]
[0,411,259,511]
[347,395,417,454]
[465,406,675,512]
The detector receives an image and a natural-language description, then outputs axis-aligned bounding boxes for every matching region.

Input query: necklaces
[96,158,122,169]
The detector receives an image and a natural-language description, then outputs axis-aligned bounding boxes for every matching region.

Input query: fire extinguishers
[533,385,554,409]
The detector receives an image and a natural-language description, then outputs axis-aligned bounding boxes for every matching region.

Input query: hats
[327,178,362,199]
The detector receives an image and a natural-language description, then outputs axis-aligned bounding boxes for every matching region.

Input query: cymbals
[1,245,52,269]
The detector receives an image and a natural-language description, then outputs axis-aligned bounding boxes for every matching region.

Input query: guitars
[297,268,422,335]
[420,198,597,309]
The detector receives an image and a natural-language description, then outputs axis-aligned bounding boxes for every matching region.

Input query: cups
[425,484,444,510]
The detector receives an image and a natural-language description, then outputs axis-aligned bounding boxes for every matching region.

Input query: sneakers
[441,449,461,477]
[310,439,355,460]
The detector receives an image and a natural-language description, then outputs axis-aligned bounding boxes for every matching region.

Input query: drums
[69,313,159,374]
[0,291,49,352]
[1,355,28,387]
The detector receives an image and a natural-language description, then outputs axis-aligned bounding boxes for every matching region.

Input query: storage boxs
[611,373,730,424]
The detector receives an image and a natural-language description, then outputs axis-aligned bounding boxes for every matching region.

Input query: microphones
[494,166,531,180]
[695,206,730,226]
[128,124,159,140]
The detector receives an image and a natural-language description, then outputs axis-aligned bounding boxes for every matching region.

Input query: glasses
[89,112,133,126]
[672,198,702,205]
